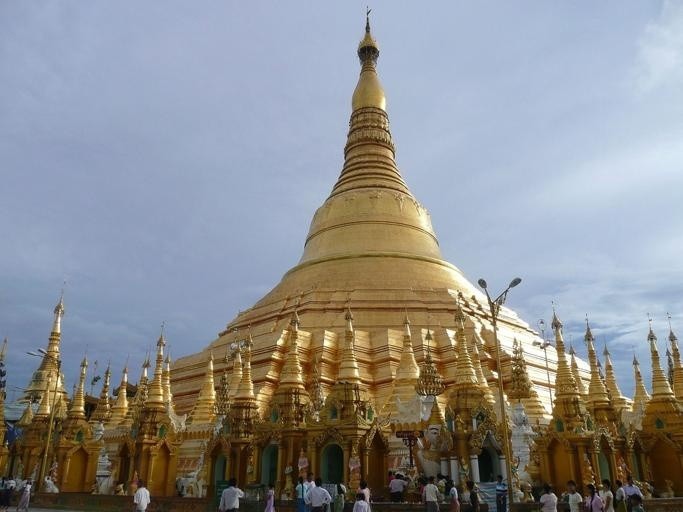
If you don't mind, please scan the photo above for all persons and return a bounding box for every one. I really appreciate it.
[0,469,657,511]
[417,423,443,480]
[48,454,58,482]
[348,446,362,490]
[297,448,309,483]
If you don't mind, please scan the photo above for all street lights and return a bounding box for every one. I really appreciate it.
[478,275,524,512]
[24,349,61,492]
[531,317,552,416]
